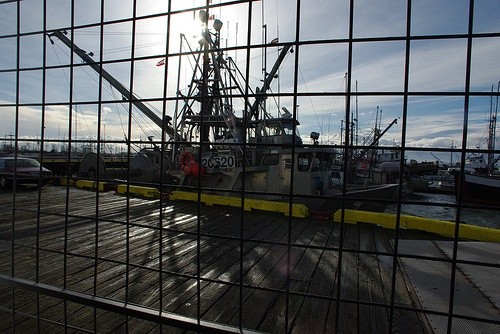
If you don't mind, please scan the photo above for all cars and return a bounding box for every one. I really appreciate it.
[0,157,51,190]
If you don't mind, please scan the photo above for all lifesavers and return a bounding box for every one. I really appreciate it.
[179,152,194,170]
[361,162,367,169]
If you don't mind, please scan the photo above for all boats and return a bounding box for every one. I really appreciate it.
[42,7,400,213]
[333,71,499,215]
